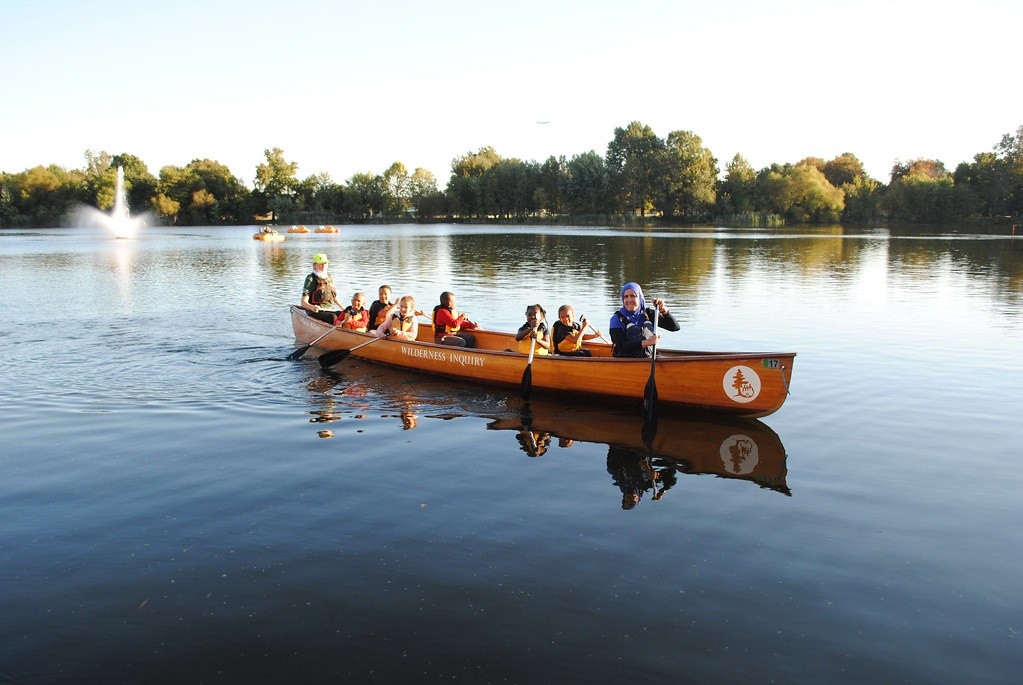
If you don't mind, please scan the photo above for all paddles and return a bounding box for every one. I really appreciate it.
[579,314,610,343]
[317,332,393,369]
[315,305,343,313]
[641,299,660,423]
[521,410,538,451]
[289,315,352,359]
[399,298,432,320]
[641,421,659,500]
[465,317,481,329]
[520,326,539,387]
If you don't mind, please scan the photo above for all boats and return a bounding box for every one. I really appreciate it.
[253,230,286,243]
[289,339,792,498]
[314,226,340,233]
[287,226,311,233]
[290,304,797,420]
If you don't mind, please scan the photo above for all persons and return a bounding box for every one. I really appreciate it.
[376,296,418,341]
[609,283,680,358]
[259,227,277,234]
[432,291,475,347]
[369,285,393,336]
[515,304,551,355]
[334,292,370,333]
[553,304,600,357]
[301,254,342,324]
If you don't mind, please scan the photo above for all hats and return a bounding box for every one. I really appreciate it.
[313,253,329,265]
